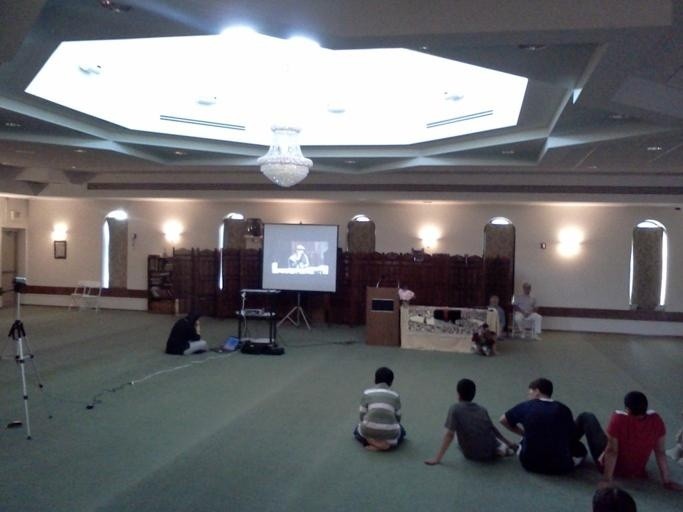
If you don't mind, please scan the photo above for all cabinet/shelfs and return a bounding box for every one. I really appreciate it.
[234,289,278,347]
[148,254,193,315]
[400,306,500,354]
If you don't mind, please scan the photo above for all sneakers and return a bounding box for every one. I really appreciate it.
[531,333,543,341]
[520,330,526,340]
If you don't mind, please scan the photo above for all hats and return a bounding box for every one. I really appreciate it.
[477,322,488,329]
[296,245,305,250]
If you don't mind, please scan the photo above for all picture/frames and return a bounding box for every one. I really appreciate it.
[54,241,67,258]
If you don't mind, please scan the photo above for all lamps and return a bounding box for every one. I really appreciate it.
[259,127,312,189]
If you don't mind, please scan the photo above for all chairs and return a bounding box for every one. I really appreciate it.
[69,281,103,314]
[511,305,534,339]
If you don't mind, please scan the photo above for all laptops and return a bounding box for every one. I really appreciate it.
[210,335,240,352]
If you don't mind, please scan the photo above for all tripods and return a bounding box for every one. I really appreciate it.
[1,319,41,438]
[276,292,313,332]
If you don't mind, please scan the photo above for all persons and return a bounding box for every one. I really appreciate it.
[471,323,500,356]
[511,282,546,343]
[570,391,683,493]
[286,244,310,268]
[589,481,637,511]
[423,378,519,466]
[352,366,405,451]
[165,308,208,355]
[499,378,576,477]
[491,295,507,340]
